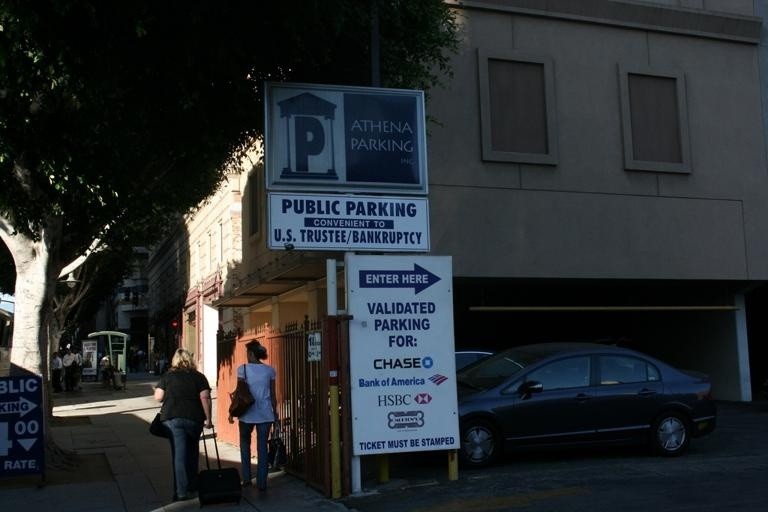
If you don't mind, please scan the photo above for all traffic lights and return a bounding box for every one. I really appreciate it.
[171,321,179,328]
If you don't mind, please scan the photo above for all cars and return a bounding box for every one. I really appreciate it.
[456,340,716,468]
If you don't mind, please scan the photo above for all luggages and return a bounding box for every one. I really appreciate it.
[198,424,242,508]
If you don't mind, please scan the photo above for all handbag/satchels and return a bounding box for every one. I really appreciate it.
[150,413,172,438]
[268,439,287,469]
[230,380,254,416]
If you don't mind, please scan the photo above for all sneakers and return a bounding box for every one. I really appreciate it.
[242,479,251,486]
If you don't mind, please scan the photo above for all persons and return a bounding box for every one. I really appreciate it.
[228,339,277,493]
[83,352,92,368]
[51,345,83,393]
[153,347,213,502]
[97,345,180,392]
